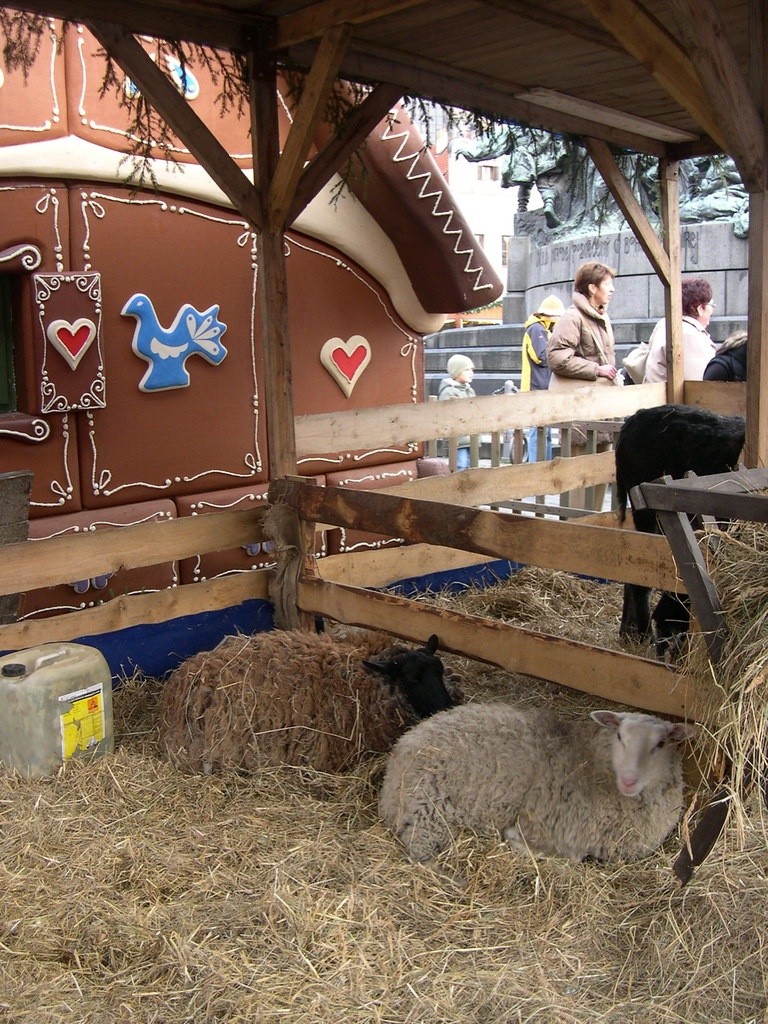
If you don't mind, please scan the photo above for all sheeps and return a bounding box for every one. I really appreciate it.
[614,400,750,661]
[164,628,703,895]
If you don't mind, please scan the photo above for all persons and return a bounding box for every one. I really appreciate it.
[454,124,566,229]
[519,296,566,464]
[546,262,616,522]
[703,331,749,383]
[438,354,479,474]
[641,278,718,385]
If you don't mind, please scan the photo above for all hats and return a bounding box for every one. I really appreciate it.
[447,354,475,380]
[538,295,565,316]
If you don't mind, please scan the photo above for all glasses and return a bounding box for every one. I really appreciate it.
[705,304,716,309]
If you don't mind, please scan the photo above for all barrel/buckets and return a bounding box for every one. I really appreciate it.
[0,642,115,778]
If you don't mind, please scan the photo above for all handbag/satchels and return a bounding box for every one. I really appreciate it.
[548,371,619,391]
[621,317,665,384]
